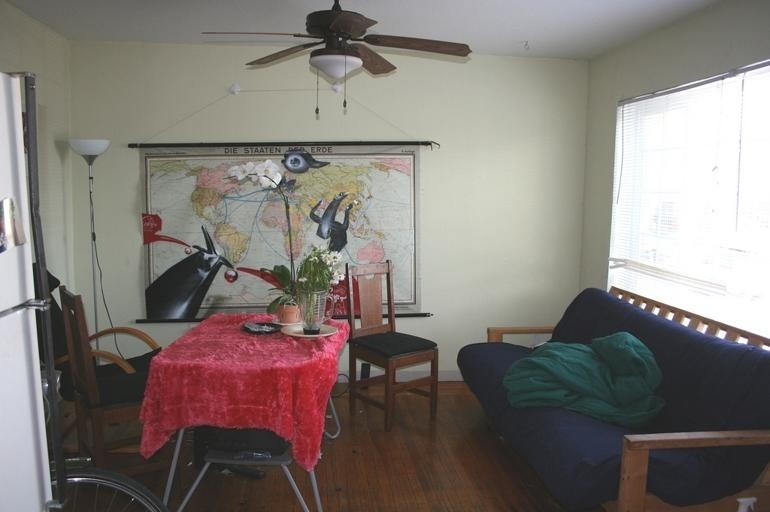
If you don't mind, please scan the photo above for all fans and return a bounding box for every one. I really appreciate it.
[199,2,473,78]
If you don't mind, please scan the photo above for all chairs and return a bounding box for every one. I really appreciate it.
[57,285,162,456]
[347,257,439,433]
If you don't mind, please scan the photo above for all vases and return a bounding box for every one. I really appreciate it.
[293,289,332,335]
[280,302,300,322]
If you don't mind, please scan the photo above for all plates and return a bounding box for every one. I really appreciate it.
[272,318,305,326]
[280,325,337,337]
[243,322,282,333]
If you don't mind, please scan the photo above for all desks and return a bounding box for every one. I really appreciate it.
[138,312,351,512]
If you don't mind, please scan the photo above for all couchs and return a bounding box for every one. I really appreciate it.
[456,283,770,512]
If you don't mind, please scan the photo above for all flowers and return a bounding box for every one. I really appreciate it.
[221,158,301,315]
[294,243,344,326]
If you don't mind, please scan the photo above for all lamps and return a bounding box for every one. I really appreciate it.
[66,137,114,366]
[307,40,366,115]
